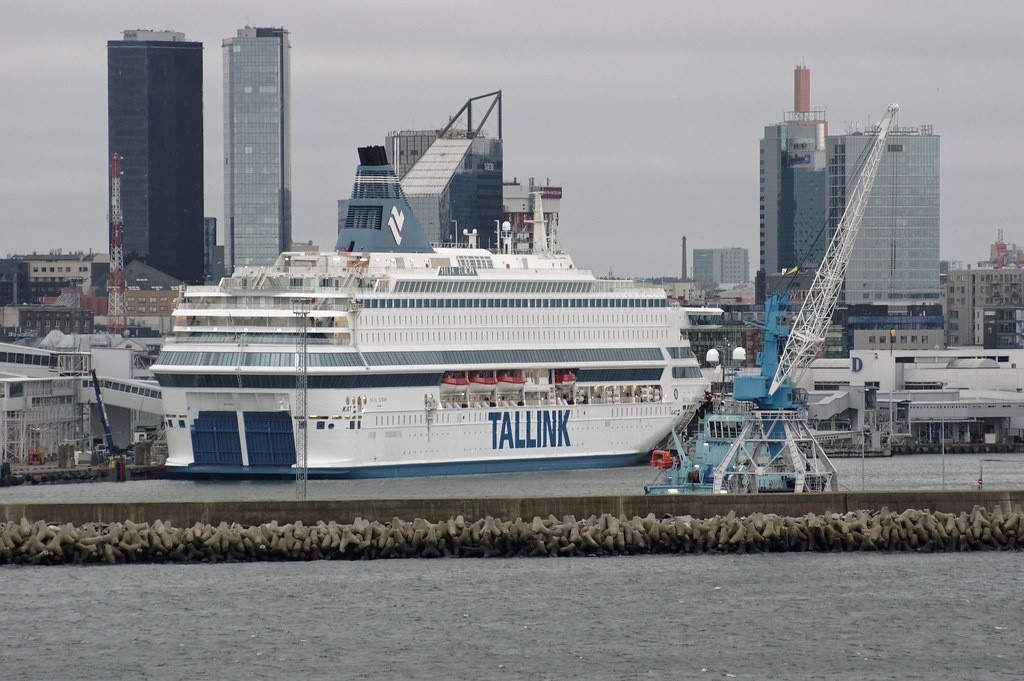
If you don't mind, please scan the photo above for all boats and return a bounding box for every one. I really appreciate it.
[467,375,499,394]
[497,375,525,394]
[439,377,469,395]
[651,449,682,470]
[549,372,578,386]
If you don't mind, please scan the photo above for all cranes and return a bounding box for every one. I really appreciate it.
[625,101,899,497]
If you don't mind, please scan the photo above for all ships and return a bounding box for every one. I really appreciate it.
[148,189,714,483]
